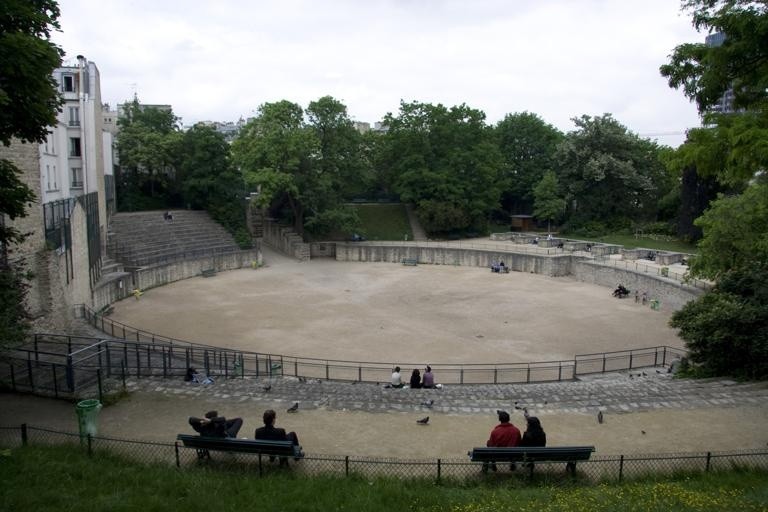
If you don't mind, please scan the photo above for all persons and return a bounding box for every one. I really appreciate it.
[486,410,521,473]
[521,417,546,467]
[253,407,305,464]
[390,366,406,389]
[422,365,434,389]
[183,365,198,382]
[410,368,421,389]
[188,408,243,440]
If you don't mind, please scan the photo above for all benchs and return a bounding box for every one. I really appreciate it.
[399,258,416,266]
[108,206,243,269]
[467,442,595,477]
[175,433,305,478]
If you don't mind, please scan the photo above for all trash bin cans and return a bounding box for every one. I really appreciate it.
[650,300,659,310]
[405,233,409,241]
[74,399,103,448]
[661,268,669,277]
[252,262,257,269]
